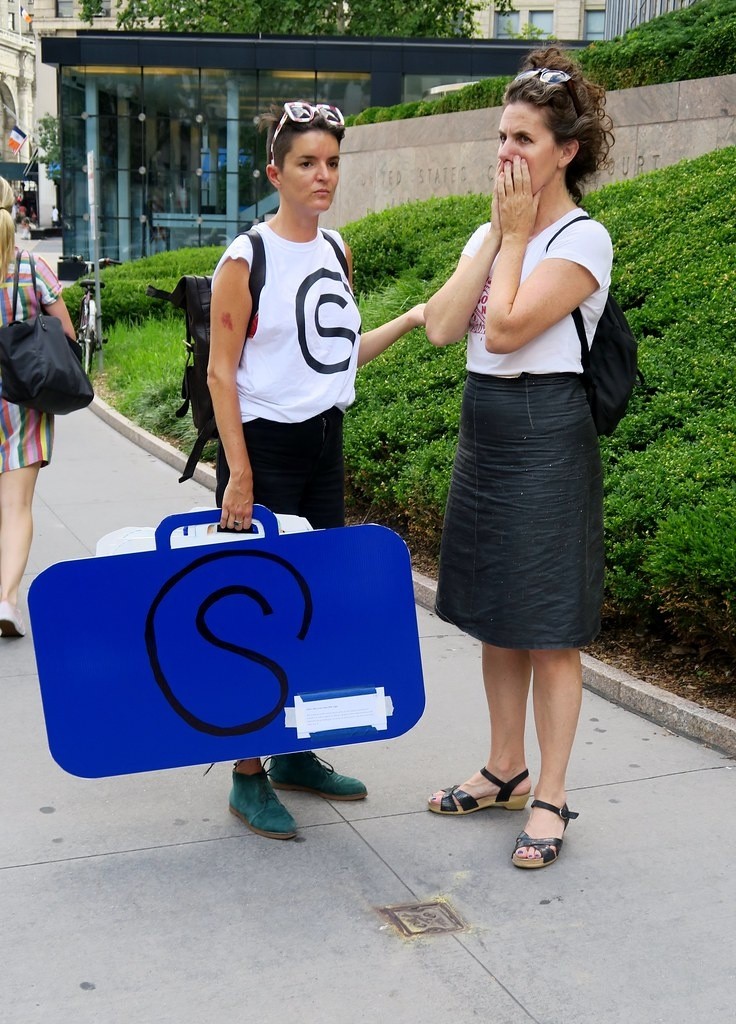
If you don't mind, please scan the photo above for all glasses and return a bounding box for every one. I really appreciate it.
[512,66,586,119]
[268,102,345,166]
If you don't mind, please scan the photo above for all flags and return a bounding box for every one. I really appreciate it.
[6,125,27,154]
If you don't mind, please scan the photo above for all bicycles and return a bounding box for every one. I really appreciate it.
[58,255,122,375]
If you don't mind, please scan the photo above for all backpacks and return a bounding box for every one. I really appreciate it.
[146,228,349,483]
[545,215,639,439]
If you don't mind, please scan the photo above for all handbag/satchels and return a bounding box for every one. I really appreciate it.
[0,249,96,415]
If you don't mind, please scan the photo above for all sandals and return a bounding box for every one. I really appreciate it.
[427,766,531,815]
[511,800,580,868]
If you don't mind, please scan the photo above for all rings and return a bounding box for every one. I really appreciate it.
[234,521,243,527]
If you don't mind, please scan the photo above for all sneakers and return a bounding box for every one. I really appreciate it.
[0,601,26,639]
[269,751,368,800]
[228,757,298,840]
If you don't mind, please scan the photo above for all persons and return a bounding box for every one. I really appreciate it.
[208,102,425,839]
[423,48,611,873]
[51,205,59,228]
[0,176,75,639]
